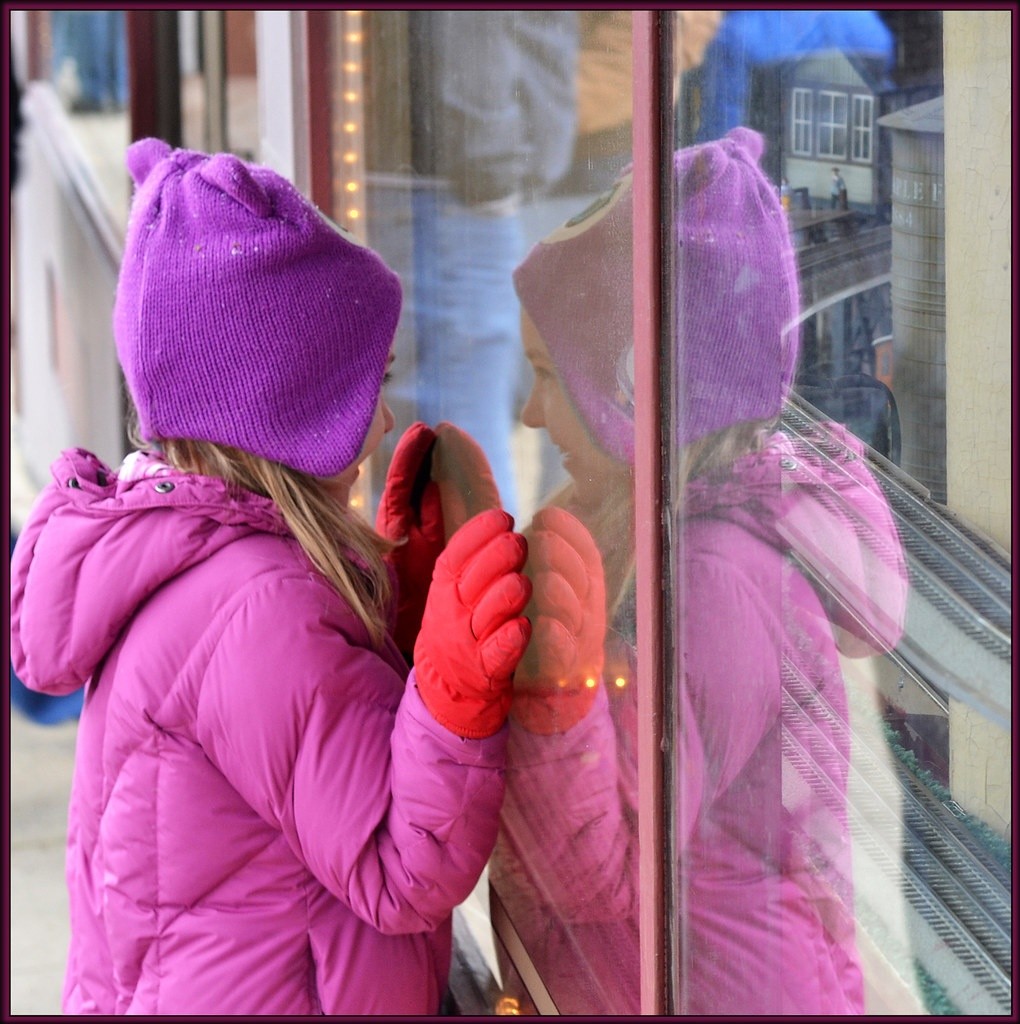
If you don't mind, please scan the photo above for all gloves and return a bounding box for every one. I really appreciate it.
[412,512,530,740]
[510,507,607,736]
[432,419,501,544]
[373,423,449,654]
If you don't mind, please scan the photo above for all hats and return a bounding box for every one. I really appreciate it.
[113,137,404,479]
[511,124,800,469]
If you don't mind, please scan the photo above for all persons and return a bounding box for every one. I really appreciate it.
[357,10,896,535]
[830,168,847,210]
[429,126,909,1016]
[778,178,792,209]
[10,136,534,1016]
